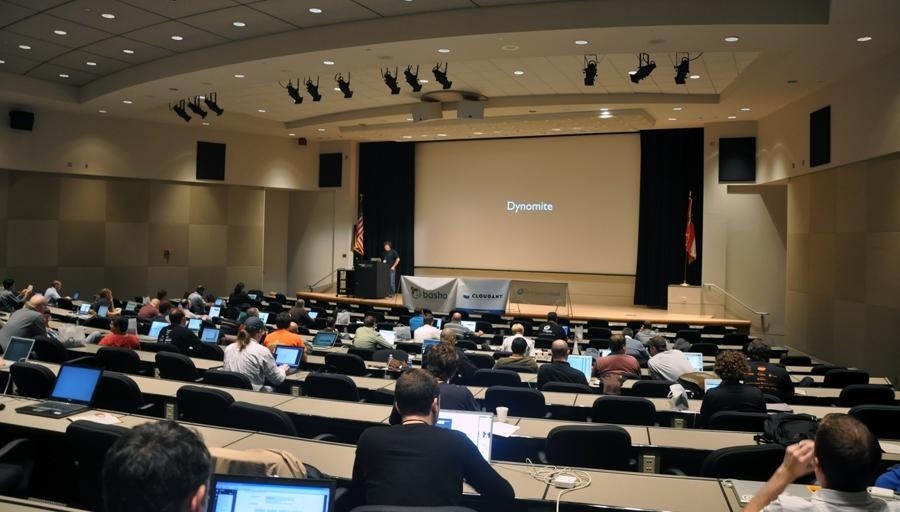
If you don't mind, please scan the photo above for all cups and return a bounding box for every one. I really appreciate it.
[495,407,509,424]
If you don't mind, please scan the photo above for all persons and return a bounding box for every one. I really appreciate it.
[99,419,215,511]
[379,240,402,296]
[352,367,515,512]
[2,275,900,428]
[740,412,900,511]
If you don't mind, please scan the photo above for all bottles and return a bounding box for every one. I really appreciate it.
[407,359,413,369]
[388,354,394,364]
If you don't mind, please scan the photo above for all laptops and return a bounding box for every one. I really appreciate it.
[703,379,746,391]
[437,410,491,469]
[16,365,104,418]
[206,475,336,512]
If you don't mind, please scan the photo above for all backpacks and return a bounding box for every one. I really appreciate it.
[753,412,819,447]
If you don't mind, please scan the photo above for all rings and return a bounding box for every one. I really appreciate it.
[798,441,803,448]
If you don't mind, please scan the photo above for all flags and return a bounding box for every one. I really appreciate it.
[350,193,365,258]
[685,190,698,264]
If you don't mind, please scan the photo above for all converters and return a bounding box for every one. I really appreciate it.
[557,475,576,489]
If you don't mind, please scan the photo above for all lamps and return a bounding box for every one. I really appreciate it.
[380,62,453,95]
[278,71,353,104]
[580,51,704,86]
[168,91,224,122]
